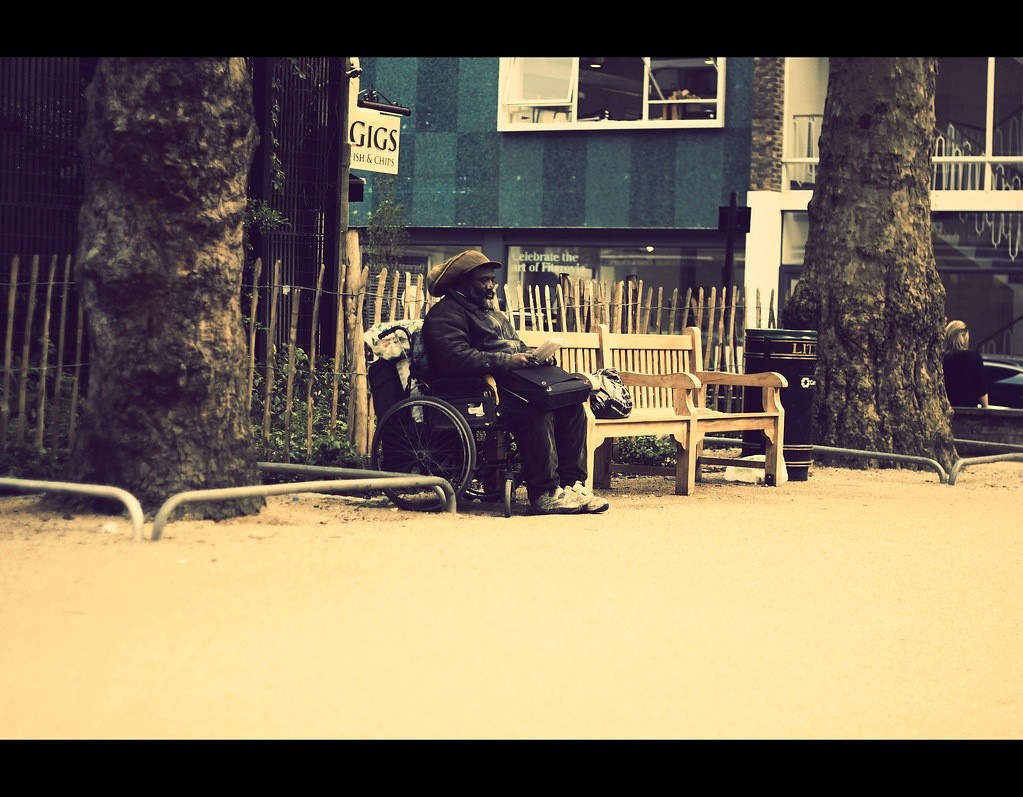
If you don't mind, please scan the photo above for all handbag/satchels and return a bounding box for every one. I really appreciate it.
[590,366,632,420]
[499,358,590,412]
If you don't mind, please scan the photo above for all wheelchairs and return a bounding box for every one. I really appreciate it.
[368,325,541,518]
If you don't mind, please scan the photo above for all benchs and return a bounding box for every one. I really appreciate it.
[485,319,788,498]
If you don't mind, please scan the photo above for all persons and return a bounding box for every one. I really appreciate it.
[944,320,988,408]
[423,250,609,514]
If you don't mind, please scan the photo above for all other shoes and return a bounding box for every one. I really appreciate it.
[531,486,583,515]
[562,481,609,514]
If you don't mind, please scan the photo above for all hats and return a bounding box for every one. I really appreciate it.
[427,249,502,298]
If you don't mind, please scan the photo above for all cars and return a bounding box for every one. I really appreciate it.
[981,355,1023,410]
